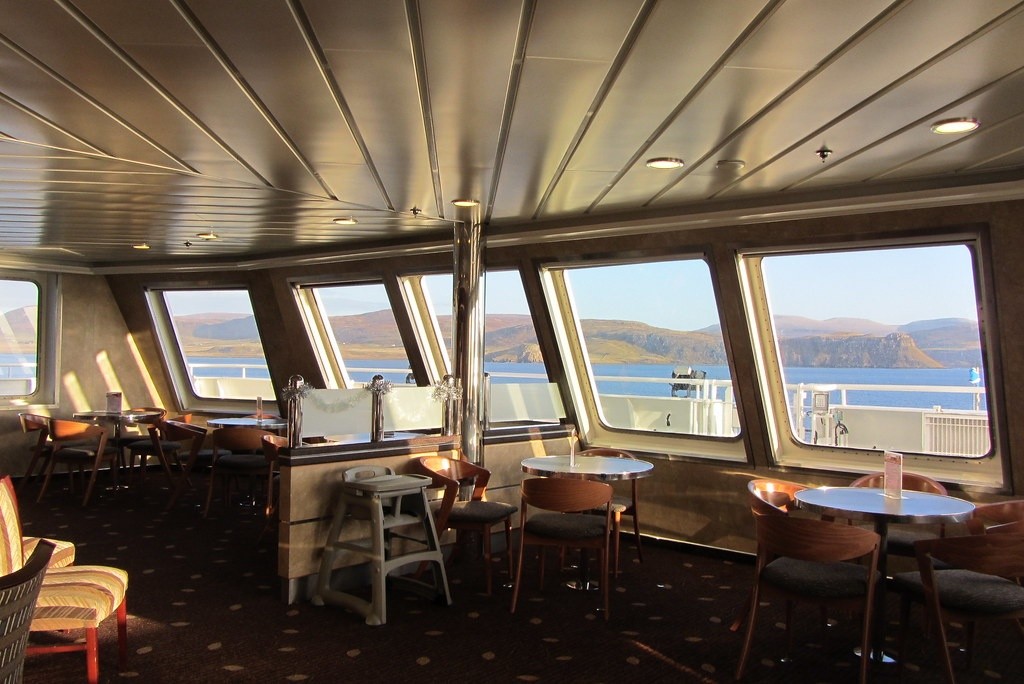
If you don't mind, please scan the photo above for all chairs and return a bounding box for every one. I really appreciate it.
[1,408,1024,684]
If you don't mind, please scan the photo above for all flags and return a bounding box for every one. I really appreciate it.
[969,367,982,384]
